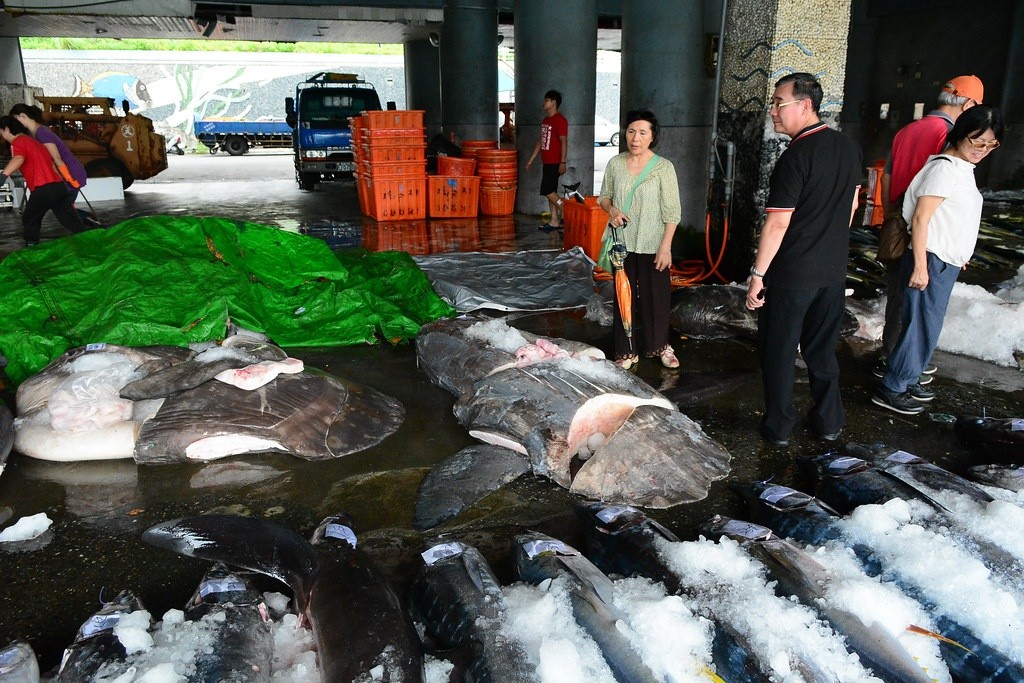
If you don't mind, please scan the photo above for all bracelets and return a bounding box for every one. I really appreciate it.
[560,162,566,163]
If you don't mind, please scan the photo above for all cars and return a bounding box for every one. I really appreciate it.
[594,114,620,147]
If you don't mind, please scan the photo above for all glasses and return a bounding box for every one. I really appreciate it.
[966,134,1001,150]
[768,97,811,115]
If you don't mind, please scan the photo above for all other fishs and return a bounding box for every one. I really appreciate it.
[0,441,1023,683]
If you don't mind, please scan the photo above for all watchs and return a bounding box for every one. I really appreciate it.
[749,263,764,277]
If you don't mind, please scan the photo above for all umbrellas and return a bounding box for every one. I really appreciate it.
[608,218,632,350]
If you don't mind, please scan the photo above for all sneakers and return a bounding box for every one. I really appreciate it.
[871,385,922,414]
[906,382,935,401]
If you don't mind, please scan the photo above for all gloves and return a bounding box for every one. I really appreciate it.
[58,165,80,189]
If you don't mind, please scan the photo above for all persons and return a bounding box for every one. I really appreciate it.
[871,104,1004,415]
[0,115,88,245]
[873,75,984,384]
[595,109,681,369]
[745,72,862,446]
[526,89,568,230]
[8,103,109,230]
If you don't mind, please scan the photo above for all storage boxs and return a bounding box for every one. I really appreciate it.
[561,195,612,262]
[346,110,482,221]
[862,160,886,227]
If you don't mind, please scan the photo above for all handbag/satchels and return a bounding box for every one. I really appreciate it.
[599,238,615,274]
[876,210,909,259]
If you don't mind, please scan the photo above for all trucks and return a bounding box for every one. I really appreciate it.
[283,71,384,192]
[192,112,295,157]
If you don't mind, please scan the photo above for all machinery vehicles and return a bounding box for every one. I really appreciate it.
[31,95,169,192]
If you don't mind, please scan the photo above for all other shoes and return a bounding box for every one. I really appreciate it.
[819,426,842,441]
[660,344,679,368]
[770,434,789,446]
[615,352,639,371]
[873,355,934,385]
[923,363,938,375]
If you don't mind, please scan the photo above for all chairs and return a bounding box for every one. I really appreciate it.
[305,100,324,120]
[348,98,366,117]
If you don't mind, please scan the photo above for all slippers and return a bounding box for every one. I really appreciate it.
[540,223,560,229]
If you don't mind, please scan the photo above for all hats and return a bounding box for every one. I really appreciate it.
[944,75,986,104]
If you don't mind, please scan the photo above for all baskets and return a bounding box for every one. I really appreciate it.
[437,139,518,216]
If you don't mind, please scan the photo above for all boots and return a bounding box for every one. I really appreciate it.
[84,213,108,228]
